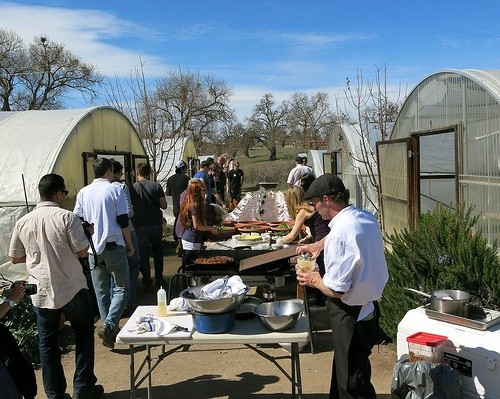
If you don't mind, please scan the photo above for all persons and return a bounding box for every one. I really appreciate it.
[9,173,104,399]
[282,185,332,306]
[296,173,389,399]
[74,158,135,349]
[286,155,314,220]
[0,283,37,399]
[110,153,244,316]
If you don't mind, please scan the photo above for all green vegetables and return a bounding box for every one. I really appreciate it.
[216,225,229,230]
[277,223,288,228]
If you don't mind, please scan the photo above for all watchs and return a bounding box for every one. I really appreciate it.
[4,299,15,308]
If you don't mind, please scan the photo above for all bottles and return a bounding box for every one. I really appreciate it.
[156,285,167,317]
[262,285,276,302]
[275,236,283,250]
[146,318,154,332]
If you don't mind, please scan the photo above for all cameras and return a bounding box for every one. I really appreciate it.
[11,281,37,295]
[80,217,89,227]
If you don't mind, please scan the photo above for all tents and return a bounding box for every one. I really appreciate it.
[307,150,327,179]
[141,137,198,190]
[322,122,394,220]
[0,105,151,283]
[376,69,500,260]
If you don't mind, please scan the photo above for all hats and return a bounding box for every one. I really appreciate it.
[175,160,190,168]
[304,172,347,201]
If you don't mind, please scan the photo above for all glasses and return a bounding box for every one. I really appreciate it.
[58,190,69,197]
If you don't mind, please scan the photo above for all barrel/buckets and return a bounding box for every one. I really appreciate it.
[406,332,448,364]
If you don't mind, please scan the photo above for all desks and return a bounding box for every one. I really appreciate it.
[116,301,313,399]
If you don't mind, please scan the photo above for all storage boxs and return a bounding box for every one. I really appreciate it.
[409,331,448,363]
[396,301,500,399]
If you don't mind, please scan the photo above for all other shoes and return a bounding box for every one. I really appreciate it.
[156,279,166,286]
[126,303,138,309]
[72,384,104,399]
[98,324,115,349]
[142,277,152,286]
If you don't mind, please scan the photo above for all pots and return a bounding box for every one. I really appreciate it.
[403,287,472,318]
[180,284,250,314]
[188,306,240,334]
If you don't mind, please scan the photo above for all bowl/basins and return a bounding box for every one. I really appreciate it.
[269,225,293,236]
[236,219,266,226]
[252,300,305,331]
[234,295,263,319]
[238,225,271,235]
[220,221,237,226]
[269,221,295,227]
[211,226,235,240]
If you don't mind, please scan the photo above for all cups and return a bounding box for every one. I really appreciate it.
[296,257,316,272]
[231,232,290,250]
[239,190,282,222]
